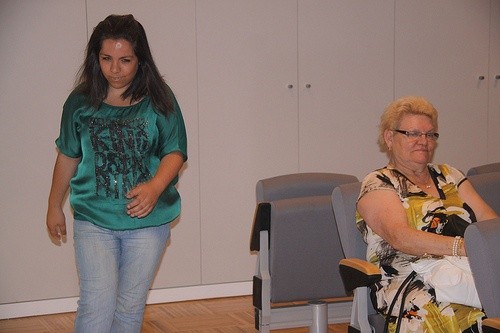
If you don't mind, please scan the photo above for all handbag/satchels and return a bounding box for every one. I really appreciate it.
[383,203,482,333]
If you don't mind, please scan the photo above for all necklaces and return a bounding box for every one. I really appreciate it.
[423,181,433,190]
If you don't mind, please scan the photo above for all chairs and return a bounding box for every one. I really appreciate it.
[248,162,500,333]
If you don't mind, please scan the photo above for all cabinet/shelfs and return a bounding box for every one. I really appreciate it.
[0,0,500,320]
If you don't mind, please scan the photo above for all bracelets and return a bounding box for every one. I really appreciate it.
[452,235,464,257]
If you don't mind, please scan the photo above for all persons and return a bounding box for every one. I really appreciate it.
[47,13,189,333]
[355,96,500,333]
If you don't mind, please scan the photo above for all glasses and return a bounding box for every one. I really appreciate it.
[395,129,439,142]
